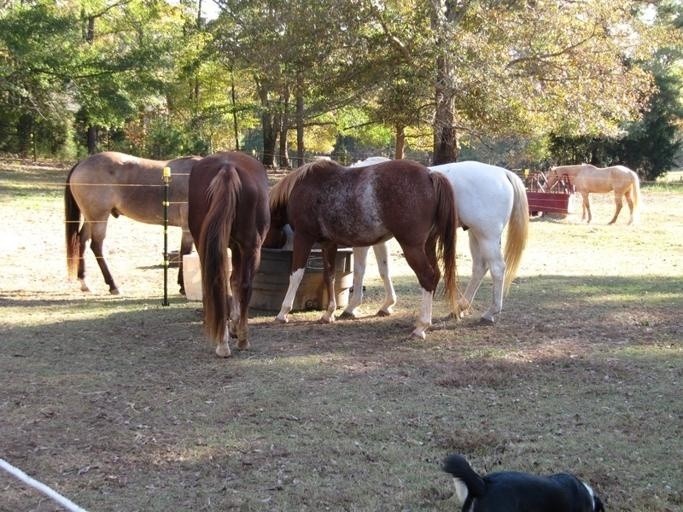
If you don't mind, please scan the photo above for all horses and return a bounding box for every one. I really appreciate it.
[263,158,474,342]
[186,150,272,358]
[335,156,530,327]
[544,165,640,225]
[64,151,204,296]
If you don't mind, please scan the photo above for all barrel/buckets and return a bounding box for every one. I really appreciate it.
[183,252,206,302]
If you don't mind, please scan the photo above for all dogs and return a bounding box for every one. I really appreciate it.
[441,452,606,512]
